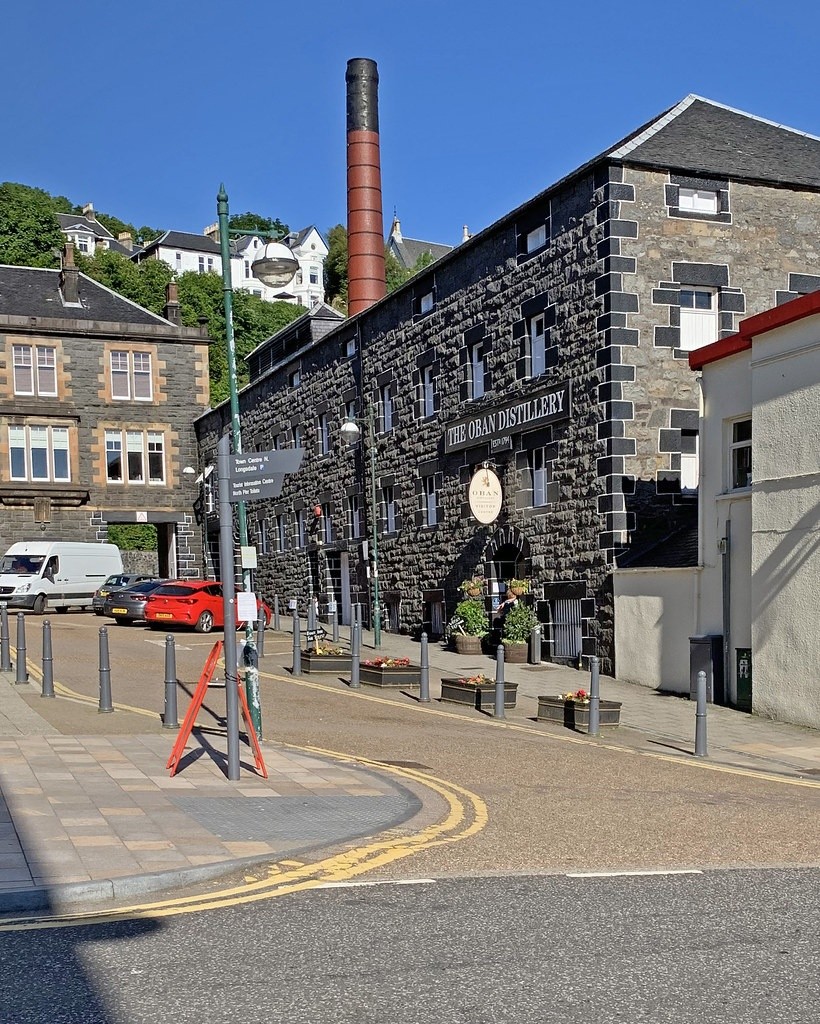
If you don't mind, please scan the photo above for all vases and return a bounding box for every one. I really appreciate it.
[537,696,622,728]
[358,662,421,689]
[439,678,519,709]
[467,589,481,595]
[300,651,353,675]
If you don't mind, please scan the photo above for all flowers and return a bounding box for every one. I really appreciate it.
[365,656,410,668]
[456,578,484,591]
[303,646,342,654]
[459,674,490,686]
[564,690,589,702]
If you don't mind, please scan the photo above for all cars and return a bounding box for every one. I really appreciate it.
[145,581,271,634]
[93,574,161,615]
[105,582,164,625]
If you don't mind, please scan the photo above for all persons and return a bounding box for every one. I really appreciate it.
[497,589,519,618]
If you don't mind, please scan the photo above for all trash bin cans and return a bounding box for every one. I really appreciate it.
[688,633,724,702]
[734,647,752,710]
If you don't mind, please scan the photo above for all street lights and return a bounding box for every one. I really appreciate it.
[214,181,301,739]
[341,407,383,650]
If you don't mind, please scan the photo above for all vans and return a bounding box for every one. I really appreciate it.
[0,542,123,613]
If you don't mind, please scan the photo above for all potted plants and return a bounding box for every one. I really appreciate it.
[510,579,530,596]
[500,598,541,664]
[451,595,490,654]
